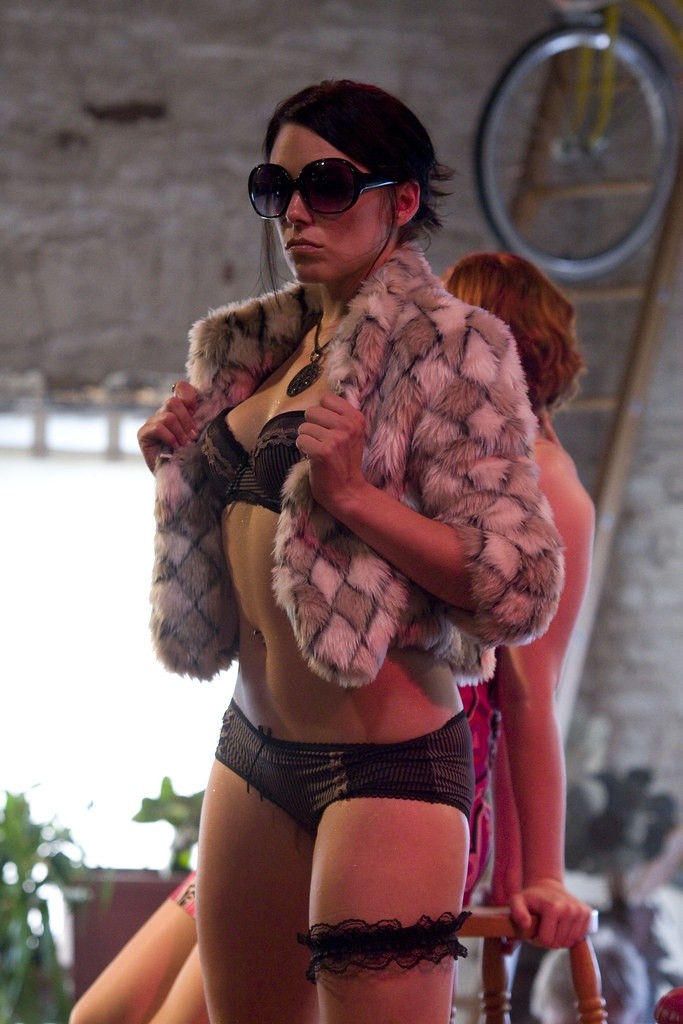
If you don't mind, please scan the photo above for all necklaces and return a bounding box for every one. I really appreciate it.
[287,317,338,398]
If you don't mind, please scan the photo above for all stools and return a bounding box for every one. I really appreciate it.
[444,907,608,1024]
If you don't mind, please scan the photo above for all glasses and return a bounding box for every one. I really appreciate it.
[248,157,407,218]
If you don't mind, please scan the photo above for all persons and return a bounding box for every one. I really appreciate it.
[136,77,569,1023]
[56,253,599,1023]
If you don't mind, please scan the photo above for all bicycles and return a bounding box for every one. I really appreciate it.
[473,0,683,283]
